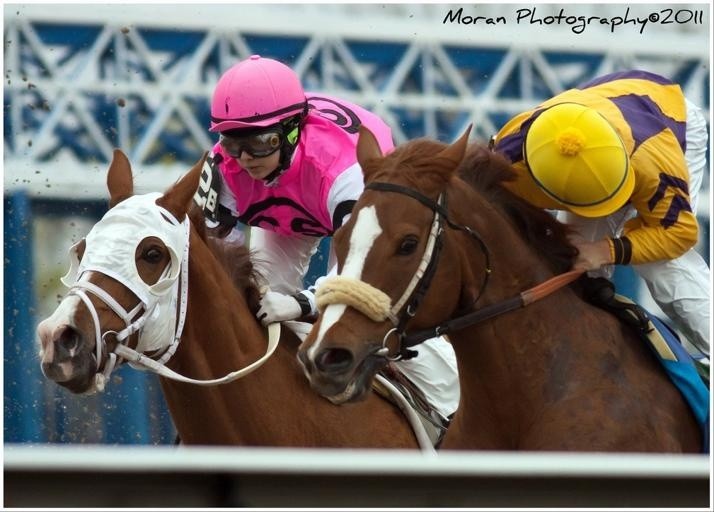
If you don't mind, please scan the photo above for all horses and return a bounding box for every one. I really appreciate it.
[37,148,449,508]
[296,122,710,508]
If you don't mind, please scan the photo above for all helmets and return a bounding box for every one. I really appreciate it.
[522,100,637,221]
[208,54,308,133]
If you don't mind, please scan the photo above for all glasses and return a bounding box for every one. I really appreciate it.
[218,126,299,158]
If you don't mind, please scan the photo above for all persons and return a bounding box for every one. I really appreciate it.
[483,68,711,361]
[187,52,461,432]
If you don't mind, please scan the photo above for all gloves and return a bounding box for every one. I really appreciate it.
[255,289,311,328]
[567,236,614,273]
